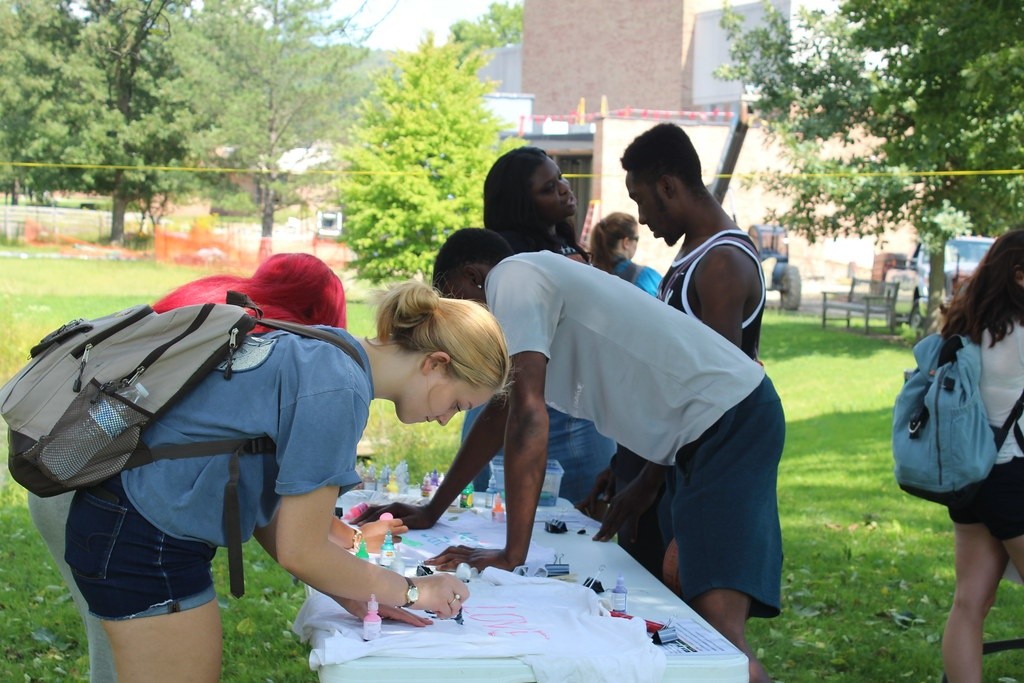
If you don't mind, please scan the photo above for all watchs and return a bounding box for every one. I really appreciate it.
[402,577,419,608]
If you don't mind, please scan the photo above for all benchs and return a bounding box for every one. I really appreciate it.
[821,276,901,335]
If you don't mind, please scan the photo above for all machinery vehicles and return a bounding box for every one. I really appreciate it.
[749,224,801,310]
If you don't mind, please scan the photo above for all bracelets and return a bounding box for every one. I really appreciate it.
[353,528,362,547]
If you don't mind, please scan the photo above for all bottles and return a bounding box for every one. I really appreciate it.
[611,572,627,613]
[421,469,445,505]
[459,482,474,508]
[455,563,471,583]
[354,529,406,578]
[356,460,410,496]
[341,502,370,522]
[39,382,151,481]
[363,594,382,641]
[484,473,505,524]
[380,512,394,522]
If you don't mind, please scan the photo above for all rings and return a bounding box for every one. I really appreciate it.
[449,599,456,605]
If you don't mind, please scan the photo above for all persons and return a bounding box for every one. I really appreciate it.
[349,227,787,683]
[942,230,1024,683]
[62,277,510,683]
[461,147,619,520]
[30,252,408,683]
[591,212,663,298]
[577,124,767,579]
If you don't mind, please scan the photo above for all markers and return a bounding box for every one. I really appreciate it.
[611,611,667,633]
[416,565,465,626]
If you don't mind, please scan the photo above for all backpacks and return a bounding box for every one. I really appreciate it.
[892,325,1024,506]
[0,291,363,497]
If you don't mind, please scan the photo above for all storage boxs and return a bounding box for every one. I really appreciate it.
[488,456,565,506]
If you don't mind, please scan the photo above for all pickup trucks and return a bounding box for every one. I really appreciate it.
[884,235,998,329]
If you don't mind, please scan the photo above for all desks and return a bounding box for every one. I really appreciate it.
[291,483,751,683]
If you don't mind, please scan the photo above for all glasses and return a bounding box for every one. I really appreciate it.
[618,235,638,244]
